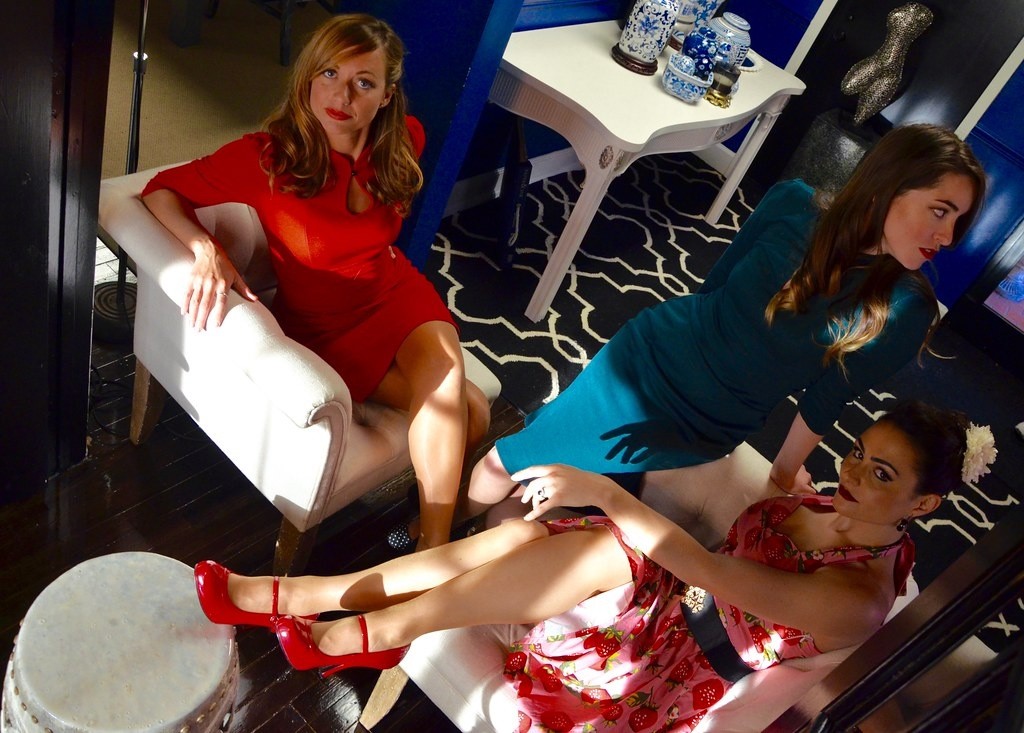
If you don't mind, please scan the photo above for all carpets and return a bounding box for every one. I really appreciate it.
[429,153,1024,655]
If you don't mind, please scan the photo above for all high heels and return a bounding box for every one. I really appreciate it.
[194,560,320,632]
[275,614,411,679]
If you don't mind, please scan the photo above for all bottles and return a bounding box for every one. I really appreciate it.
[696,32,718,59]
[682,27,709,60]
[712,41,731,65]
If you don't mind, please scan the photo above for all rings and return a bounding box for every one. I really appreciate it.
[538,486,547,499]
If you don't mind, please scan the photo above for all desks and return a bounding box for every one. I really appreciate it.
[494,13,806,324]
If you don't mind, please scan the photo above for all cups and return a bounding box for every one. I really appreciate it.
[704,62,742,108]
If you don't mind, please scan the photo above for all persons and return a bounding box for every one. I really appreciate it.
[385,125,988,552]
[136,15,489,553]
[195,398,997,733]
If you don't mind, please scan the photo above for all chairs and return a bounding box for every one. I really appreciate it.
[350,443,1024,733]
[0,554,238,733]
[94,158,502,573]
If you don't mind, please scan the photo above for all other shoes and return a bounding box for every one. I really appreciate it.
[386,514,419,551]
